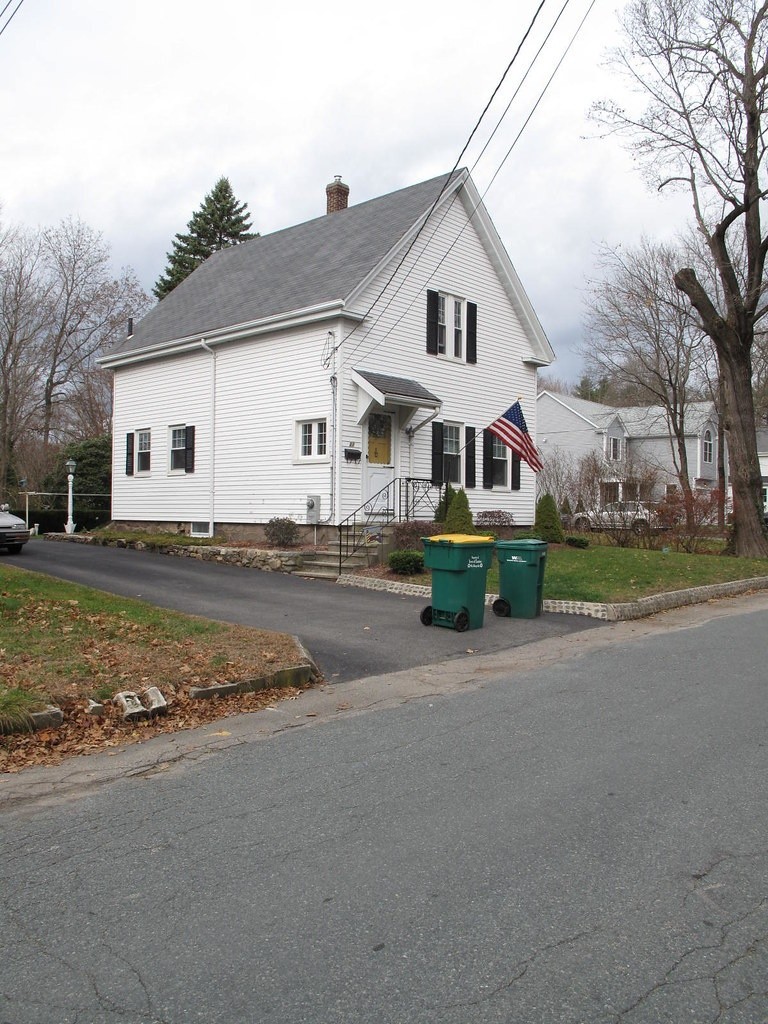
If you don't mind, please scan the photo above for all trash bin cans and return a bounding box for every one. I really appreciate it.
[420,534,497,632]
[491,539,548,620]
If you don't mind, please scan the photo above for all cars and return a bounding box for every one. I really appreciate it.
[567,501,675,536]
[0,503,31,554]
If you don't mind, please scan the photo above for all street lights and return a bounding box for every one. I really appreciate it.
[65,457,77,535]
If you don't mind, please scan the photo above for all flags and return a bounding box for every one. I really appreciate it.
[482,400,543,473]
